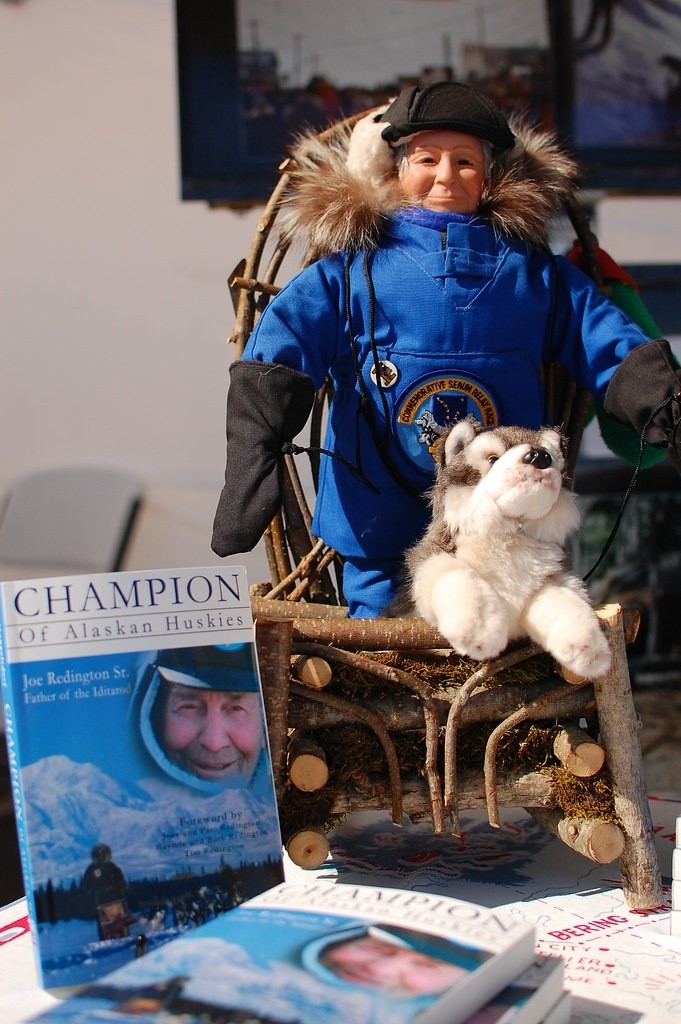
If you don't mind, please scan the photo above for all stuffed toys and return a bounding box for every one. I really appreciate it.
[393,415,614,680]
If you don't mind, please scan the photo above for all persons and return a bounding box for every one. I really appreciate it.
[78,842,131,941]
[129,642,271,799]
[297,920,483,1004]
[208,81,681,618]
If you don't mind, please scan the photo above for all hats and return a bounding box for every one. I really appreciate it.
[154,642,260,692]
[365,922,490,974]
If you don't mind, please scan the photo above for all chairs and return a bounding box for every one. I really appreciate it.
[225,97,664,915]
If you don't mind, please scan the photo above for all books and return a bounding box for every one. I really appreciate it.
[0,566,286,989]
[31,879,574,1024]
